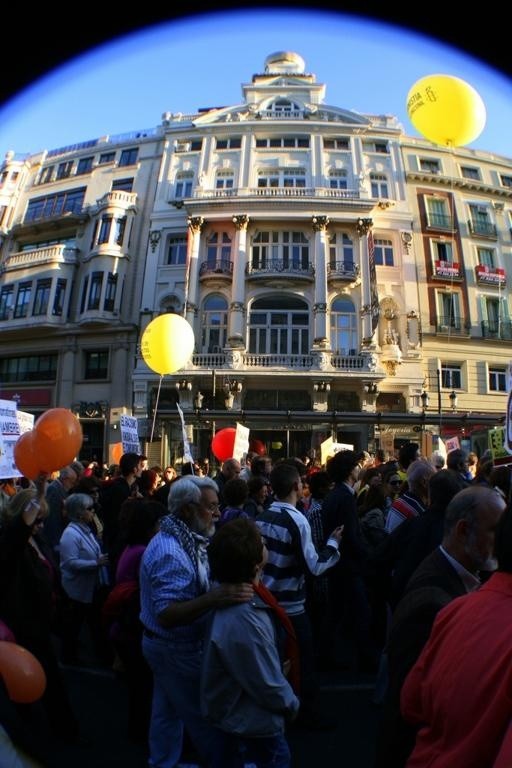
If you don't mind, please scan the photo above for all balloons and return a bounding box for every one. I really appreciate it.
[30,406,86,473]
[404,72,489,149]
[139,312,196,378]
[11,429,49,482]
[209,425,238,463]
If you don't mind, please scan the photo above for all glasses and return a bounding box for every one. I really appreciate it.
[86,505,93,511]
[390,480,403,486]
[34,517,44,525]
[261,535,267,546]
[207,501,222,512]
